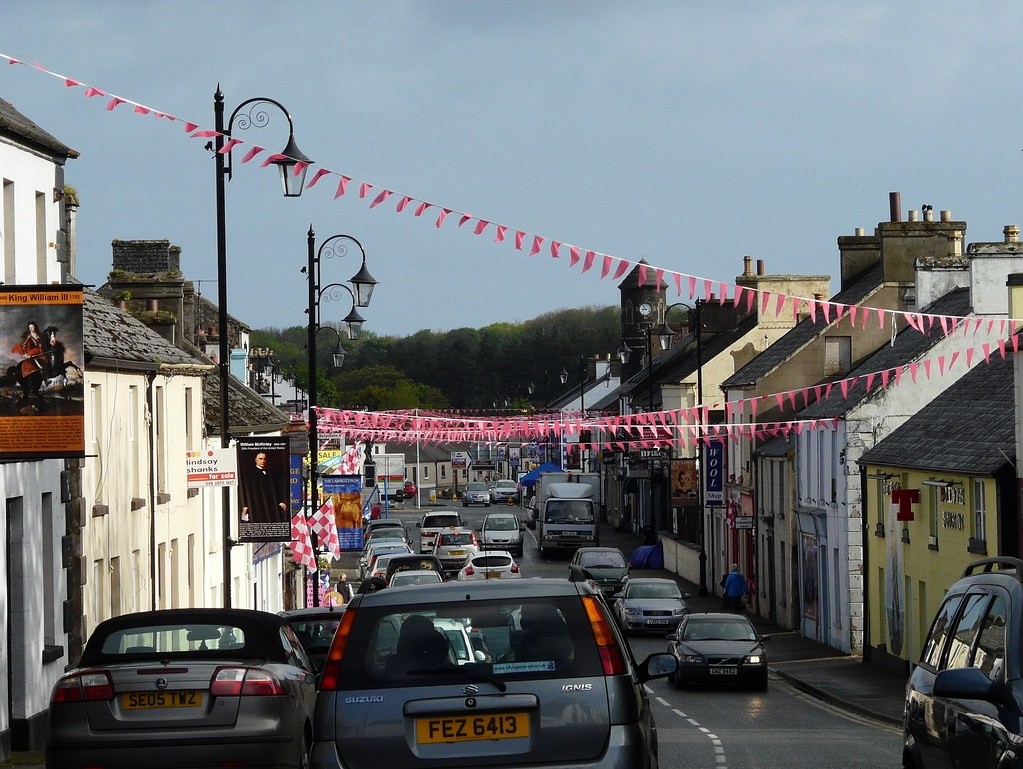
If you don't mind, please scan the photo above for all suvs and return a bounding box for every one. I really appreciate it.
[901,557,1023,769]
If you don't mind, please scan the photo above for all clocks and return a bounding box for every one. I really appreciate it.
[639,302,652,316]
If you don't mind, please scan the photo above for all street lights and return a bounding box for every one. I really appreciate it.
[257,354,273,396]
[206,80,315,647]
[271,361,296,405]
[602,359,631,534]
[527,368,549,462]
[618,322,657,546]
[560,351,585,475]
[298,221,381,640]
[657,302,708,597]
[503,391,523,471]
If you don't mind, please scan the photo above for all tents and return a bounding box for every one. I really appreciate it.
[519,461,565,520]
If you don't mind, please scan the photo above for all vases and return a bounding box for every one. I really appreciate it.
[207,326,216,336]
[146,300,159,311]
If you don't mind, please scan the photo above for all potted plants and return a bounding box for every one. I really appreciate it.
[110,290,133,309]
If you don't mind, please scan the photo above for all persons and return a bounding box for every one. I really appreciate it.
[21,321,42,349]
[334,572,354,604]
[240,452,287,523]
[725,564,749,614]
[673,471,692,497]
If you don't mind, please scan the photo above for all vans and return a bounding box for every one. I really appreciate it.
[402,480,416,499]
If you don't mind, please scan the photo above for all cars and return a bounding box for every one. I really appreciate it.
[462,479,520,507]
[275,510,526,674]
[666,612,772,691]
[524,496,537,530]
[569,547,634,598]
[45,607,322,769]
[613,577,693,637]
[308,566,682,769]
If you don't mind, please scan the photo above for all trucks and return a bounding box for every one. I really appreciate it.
[371,452,407,502]
[532,472,602,560]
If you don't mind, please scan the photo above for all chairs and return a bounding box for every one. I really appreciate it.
[515,626,573,661]
[385,650,447,680]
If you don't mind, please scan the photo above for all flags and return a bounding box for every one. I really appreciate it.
[332,444,360,474]
[307,497,341,561]
[284,508,317,574]
